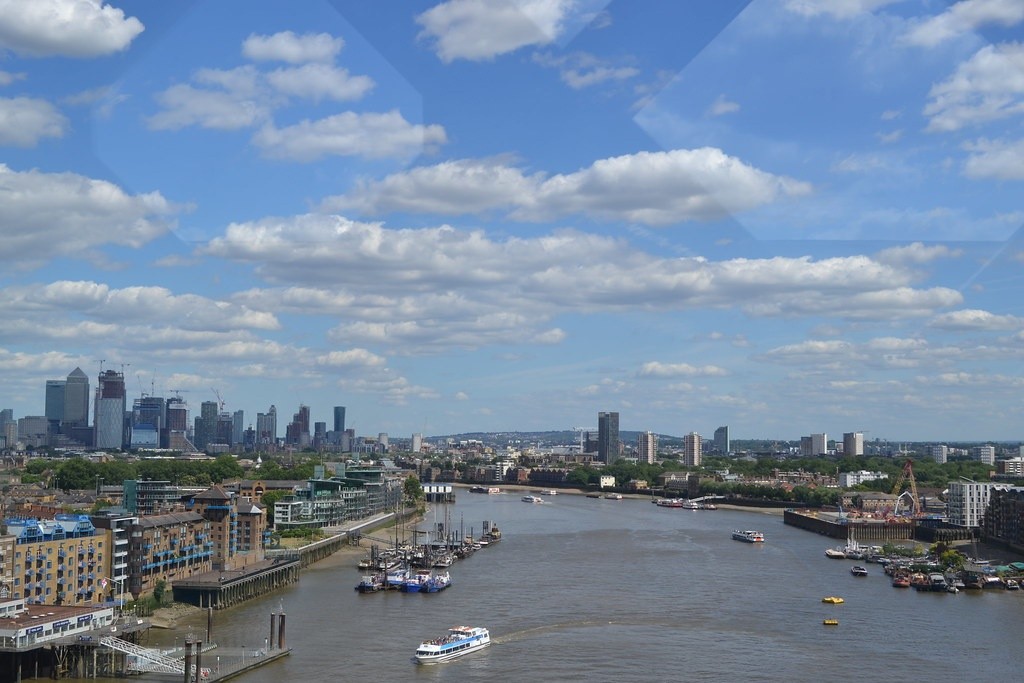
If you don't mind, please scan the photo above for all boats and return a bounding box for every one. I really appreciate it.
[825,548,846,559]
[732,529,764,542]
[605,492,622,500]
[824,619,838,625]
[470,485,489,493]
[353,519,503,593]
[851,565,868,575]
[415,625,491,665]
[867,553,1024,593]
[521,495,544,503]
[541,489,559,495]
[656,498,718,510]
[822,597,844,603]
